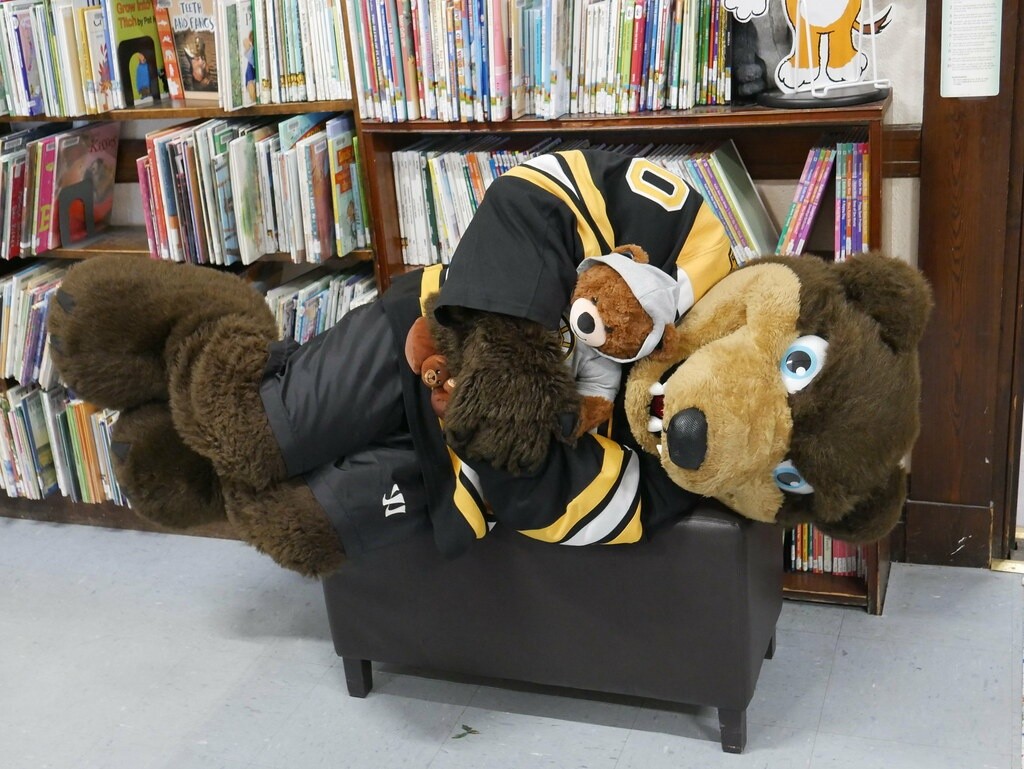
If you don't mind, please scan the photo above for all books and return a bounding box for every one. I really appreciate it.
[782,523,868,581]
[134,114,372,268]
[0,0,355,118]
[0,121,123,261]
[391,133,589,267]
[345,0,734,124]
[0,261,75,390]
[774,126,870,267]
[0,382,133,511]
[236,260,378,347]
[587,138,779,268]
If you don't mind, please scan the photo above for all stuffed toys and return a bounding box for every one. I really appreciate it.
[49,146,931,574]
[401,243,700,440]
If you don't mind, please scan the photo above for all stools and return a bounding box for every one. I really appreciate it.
[320,498,785,757]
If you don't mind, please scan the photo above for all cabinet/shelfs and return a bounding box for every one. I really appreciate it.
[357,108,902,619]
[0,0,381,541]
